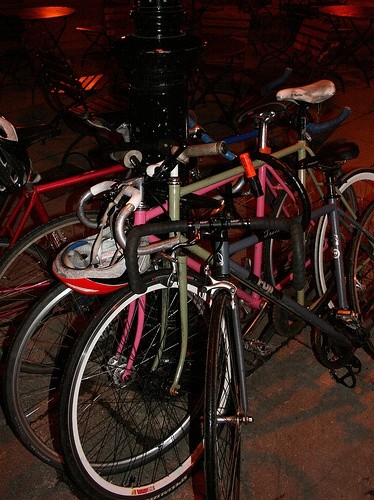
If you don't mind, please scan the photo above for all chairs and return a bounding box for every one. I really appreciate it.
[1,0,374,135]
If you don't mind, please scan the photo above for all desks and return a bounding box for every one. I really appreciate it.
[183,36,247,131]
[18,5,77,105]
[320,5,374,87]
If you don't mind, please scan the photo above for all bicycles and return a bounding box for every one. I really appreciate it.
[0,78,374,500]
